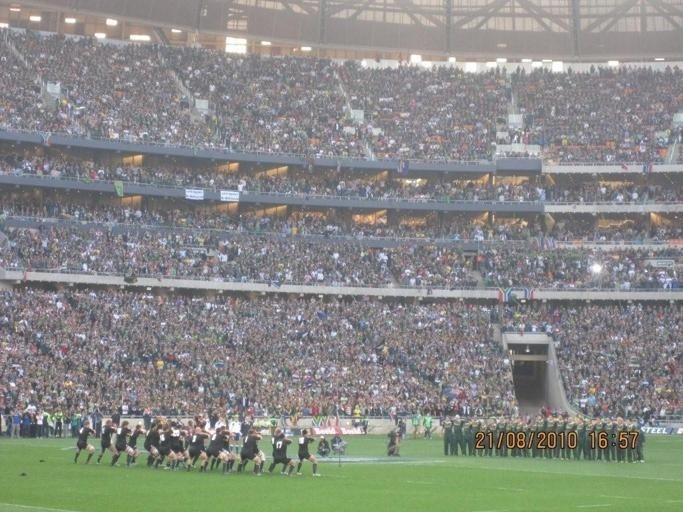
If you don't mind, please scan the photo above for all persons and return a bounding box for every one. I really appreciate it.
[0,28,682,476]
